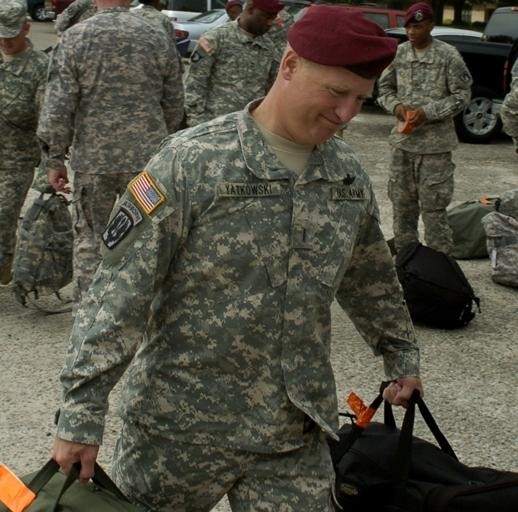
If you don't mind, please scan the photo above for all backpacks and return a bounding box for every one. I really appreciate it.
[392,241,483,332]
[9,177,76,305]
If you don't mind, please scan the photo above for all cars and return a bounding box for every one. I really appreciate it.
[27,1,75,22]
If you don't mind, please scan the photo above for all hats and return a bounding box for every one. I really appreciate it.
[0,0,32,40]
[285,3,399,81]
[252,0,286,18]
[402,2,436,29]
[224,0,243,14]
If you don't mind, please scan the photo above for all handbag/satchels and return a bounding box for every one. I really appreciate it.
[443,187,518,290]
[327,377,518,512]
[0,449,146,512]
